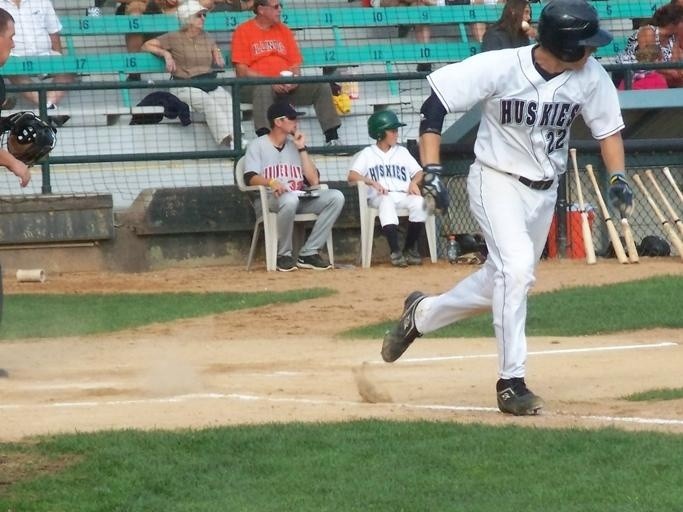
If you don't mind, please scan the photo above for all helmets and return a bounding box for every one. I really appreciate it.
[367,111,407,139]
[641,236,670,256]
[7,112,57,166]
[455,232,488,255]
[538,0,613,62]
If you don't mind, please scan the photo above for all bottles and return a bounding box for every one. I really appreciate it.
[349,72,360,99]
[445,236,461,264]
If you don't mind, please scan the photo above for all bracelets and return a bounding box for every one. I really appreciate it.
[268,178,277,187]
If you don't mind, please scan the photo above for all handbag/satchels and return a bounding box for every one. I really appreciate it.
[189,72,218,91]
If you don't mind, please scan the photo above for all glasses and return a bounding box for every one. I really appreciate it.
[196,13,207,17]
[260,4,283,9]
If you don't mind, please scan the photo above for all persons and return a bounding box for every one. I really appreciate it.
[0,7,57,379]
[361,0,683,90]
[380,0,634,416]
[244,102,345,273]
[0,0,349,159]
[348,109,426,268]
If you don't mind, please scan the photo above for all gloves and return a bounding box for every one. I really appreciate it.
[607,173,634,218]
[420,164,450,216]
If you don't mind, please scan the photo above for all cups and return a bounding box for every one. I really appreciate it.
[14,268,46,285]
[279,70,293,87]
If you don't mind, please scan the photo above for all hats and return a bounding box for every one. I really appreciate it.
[267,101,305,120]
[176,0,207,17]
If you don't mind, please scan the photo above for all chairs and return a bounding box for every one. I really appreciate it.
[358,180,437,269]
[235,155,335,272]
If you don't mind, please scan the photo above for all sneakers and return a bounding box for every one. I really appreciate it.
[381,290,426,363]
[325,139,349,157]
[406,248,421,264]
[497,379,545,415]
[391,251,408,267]
[296,254,332,271]
[277,256,298,272]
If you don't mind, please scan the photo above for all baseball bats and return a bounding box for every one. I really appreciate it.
[570,148,596,265]
[663,166,683,201]
[621,208,639,263]
[646,170,682,234]
[632,173,683,260]
[584,164,627,264]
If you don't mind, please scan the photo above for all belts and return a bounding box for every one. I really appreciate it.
[511,173,554,190]
